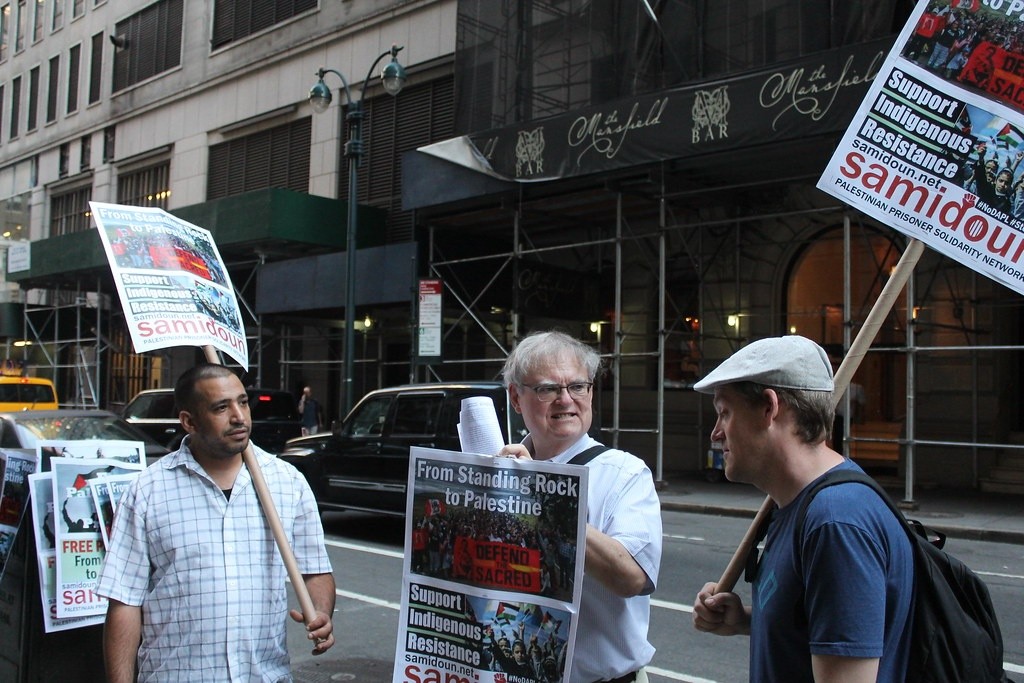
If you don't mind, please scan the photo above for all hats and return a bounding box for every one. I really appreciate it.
[693,334,836,395]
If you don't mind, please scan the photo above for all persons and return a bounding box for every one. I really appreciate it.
[902,1,1023,221]
[498,329,666,683]
[689,332,916,683]
[97,363,339,683]
[299,386,323,437]
[2,443,143,552]
[106,224,242,332]
[412,491,576,682]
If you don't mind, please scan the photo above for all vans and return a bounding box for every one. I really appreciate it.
[0,376,58,415]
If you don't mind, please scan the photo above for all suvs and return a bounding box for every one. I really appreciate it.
[114,388,301,459]
[282,381,520,530]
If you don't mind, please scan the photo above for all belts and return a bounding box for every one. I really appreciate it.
[607,671,636,683]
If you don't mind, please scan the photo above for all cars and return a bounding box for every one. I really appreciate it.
[0,409,173,533]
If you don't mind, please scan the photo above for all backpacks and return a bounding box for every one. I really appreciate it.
[792,469,1010,683]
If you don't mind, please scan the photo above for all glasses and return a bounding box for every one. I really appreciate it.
[746,510,771,584]
[517,380,597,403]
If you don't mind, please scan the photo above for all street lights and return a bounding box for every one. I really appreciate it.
[309,44,408,434]
